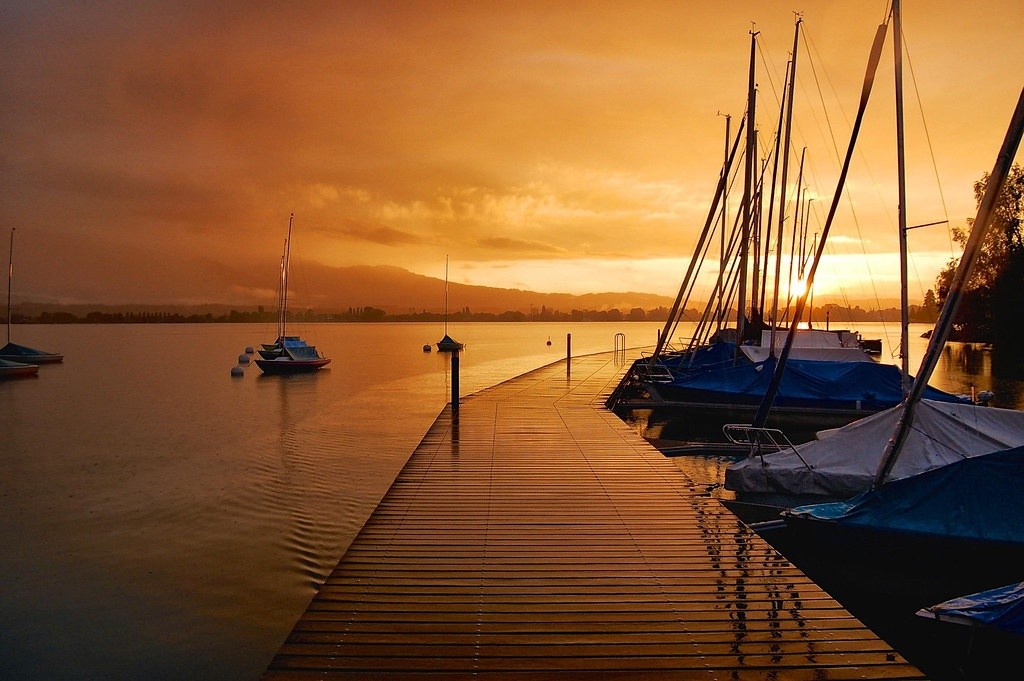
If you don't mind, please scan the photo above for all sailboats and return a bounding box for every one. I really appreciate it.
[0,226,64,365]
[620,0,1024,652]
[436,254,465,351]
[253,213,331,374]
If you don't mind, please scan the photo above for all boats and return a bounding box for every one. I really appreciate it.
[0,358,40,377]
